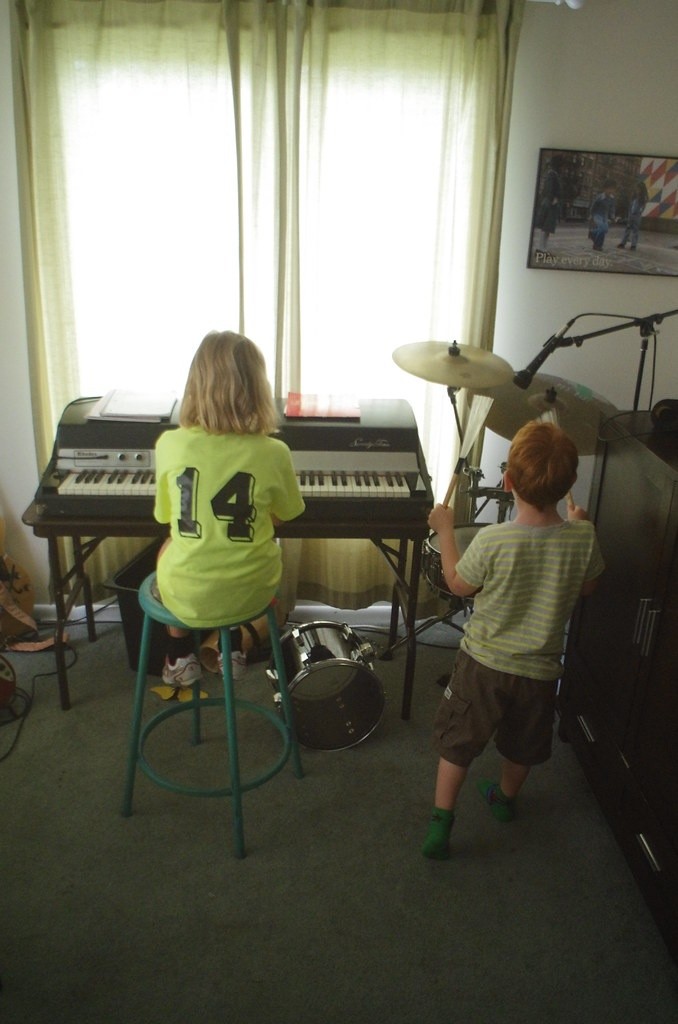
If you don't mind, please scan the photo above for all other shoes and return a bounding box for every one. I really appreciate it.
[631,246,636,249]
[594,244,602,250]
[617,244,624,249]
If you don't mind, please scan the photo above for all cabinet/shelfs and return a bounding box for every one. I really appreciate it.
[559,412,678,960]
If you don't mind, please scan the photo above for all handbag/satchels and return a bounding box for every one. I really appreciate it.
[588,215,598,241]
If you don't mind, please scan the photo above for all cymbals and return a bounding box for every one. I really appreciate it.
[467,373,629,455]
[392,341,513,388]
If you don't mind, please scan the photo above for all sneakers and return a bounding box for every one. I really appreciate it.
[163,653,201,686]
[216,641,246,680]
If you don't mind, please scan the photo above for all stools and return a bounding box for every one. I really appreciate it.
[119,571,303,858]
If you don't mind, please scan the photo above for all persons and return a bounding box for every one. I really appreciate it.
[419,421,606,860]
[536,154,567,256]
[153,330,306,687]
[617,182,649,251]
[588,177,618,251]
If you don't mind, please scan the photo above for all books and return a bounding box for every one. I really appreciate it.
[83,388,177,423]
[284,391,362,418]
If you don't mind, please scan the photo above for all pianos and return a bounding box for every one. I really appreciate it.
[21,393,434,538]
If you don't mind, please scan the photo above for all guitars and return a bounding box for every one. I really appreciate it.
[0,517,35,640]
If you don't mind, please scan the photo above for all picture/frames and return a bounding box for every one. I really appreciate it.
[526,148,678,277]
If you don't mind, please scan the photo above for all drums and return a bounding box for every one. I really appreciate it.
[420,520,498,598]
[268,620,385,752]
